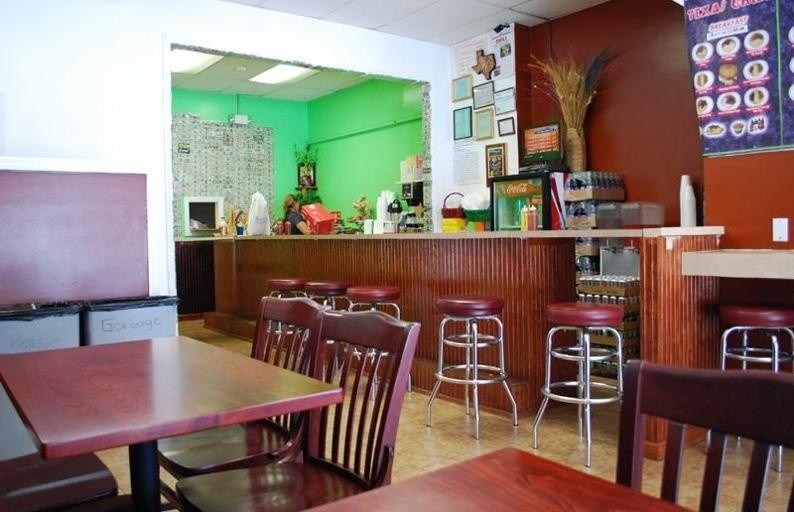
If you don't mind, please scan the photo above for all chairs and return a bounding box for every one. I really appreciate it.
[181,305,422,512]
[0,429,136,511]
[159,288,345,512]
[611,345,794,512]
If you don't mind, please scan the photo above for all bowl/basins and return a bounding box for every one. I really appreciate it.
[441,207,467,233]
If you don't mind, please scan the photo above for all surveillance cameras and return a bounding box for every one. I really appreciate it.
[494,24,502,33]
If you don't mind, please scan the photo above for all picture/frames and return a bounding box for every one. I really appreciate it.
[484,143,510,190]
[497,115,515,137]
[492,86,517,116]
[471,107,495,140]
[295,163,316,187]
[471,82,495,110]
[450,75,473,104]
[452,107,472,140]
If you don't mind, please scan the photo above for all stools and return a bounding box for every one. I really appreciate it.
[422,290,521,443]
[259,278,306,363]
[531,302,632,468]
[340,285,413,401]
[704,299,794,473]
[297,279,348,387]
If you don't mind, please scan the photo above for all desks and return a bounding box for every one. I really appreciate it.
[0,335,345,512]
[291,439,695,512]
[673,245,794,284]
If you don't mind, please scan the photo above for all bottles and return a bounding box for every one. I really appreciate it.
[519,206,539,230]
[568,199,616,216]
[563,170,624,191]
[680,175,698,227]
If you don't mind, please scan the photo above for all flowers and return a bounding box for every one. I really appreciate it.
[344,193,372,225]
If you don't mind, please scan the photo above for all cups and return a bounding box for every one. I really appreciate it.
[237,224,245,236]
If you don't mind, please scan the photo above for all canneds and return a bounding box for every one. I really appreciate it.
[575,276,639,374]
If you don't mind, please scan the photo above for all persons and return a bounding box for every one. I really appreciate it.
[281,194,311,235]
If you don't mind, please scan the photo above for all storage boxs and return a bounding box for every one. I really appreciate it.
[302,203,333,234]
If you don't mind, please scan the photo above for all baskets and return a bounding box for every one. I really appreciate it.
[463,209,490,221]
[442,192,466,218]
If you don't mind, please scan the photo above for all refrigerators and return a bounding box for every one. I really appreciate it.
[490,171,566,230]
